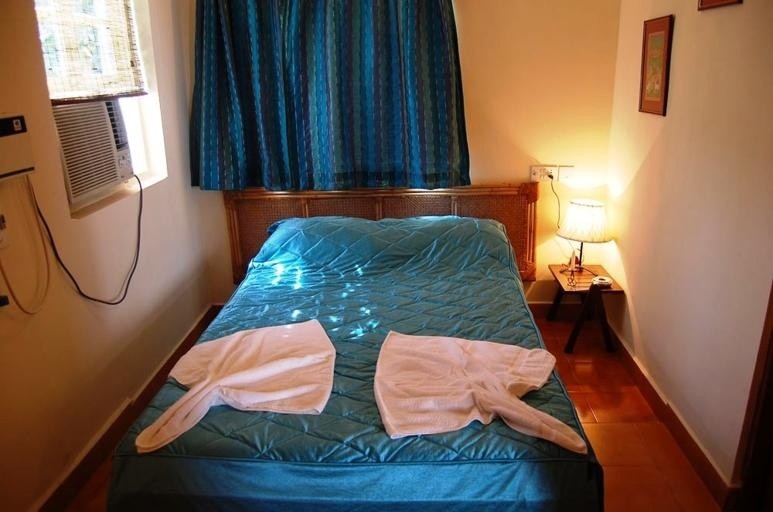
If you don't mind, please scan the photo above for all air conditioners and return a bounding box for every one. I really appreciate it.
[50,95,137,217]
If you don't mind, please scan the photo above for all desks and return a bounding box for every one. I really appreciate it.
[549,264,624,355]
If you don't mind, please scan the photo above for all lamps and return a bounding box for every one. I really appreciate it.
[555,198,616,276]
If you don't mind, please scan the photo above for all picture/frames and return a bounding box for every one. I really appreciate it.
[638,14,675,117]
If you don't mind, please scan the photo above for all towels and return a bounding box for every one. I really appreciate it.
[373,327,589,457]
[133,319,337,456]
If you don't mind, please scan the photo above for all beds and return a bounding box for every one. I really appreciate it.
[111,182,604,510]
